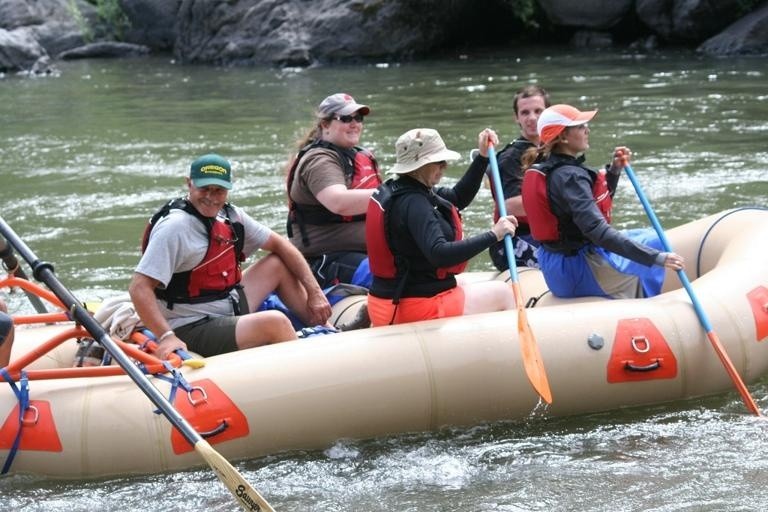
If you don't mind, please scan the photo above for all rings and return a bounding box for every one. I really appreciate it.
[675,260,679,265]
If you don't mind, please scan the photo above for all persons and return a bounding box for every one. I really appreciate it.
[285,93,384,289]
[484,85,552,272]
[366,127,520,328]
[520,104,687,300]
[0,234,15,371]
[128,155,343,361]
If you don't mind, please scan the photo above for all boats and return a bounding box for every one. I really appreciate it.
[0,207,767,483]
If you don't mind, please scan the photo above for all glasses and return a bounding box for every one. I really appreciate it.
[326,114,364,123]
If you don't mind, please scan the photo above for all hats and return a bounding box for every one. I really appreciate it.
[387,127,461,174]
[190,154,231,191]
[316,93,369,119]
[536,104,597,144]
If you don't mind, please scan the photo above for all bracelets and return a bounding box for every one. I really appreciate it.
[156,330,175,343]
[655,252,667,268]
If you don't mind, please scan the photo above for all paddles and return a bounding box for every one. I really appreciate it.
[0,213,276,512]
[616,150,763,416]
[487,140,554,406]
[82,301,204,369]
